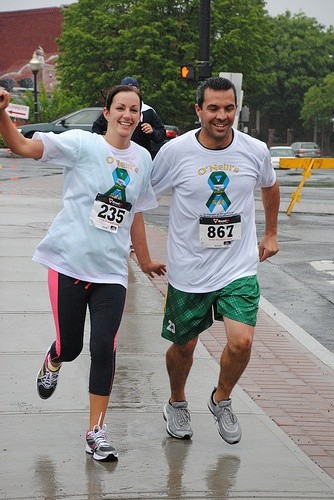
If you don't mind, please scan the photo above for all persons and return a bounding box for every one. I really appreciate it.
[0,85,168,461]
[92,77,166,161]
[152,77,280,445]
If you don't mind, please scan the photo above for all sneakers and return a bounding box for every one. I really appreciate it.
[36,344,62,400]
[207,386,242,445]
[85,411,119,462]
[163,398,194,440]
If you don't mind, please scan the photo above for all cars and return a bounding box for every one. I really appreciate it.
[17,107,179,158]
[291,141,322,158]
[269,145,297,169]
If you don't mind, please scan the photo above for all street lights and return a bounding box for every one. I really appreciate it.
[29,56,41,123]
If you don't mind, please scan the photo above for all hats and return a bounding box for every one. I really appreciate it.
[121,77,141,89]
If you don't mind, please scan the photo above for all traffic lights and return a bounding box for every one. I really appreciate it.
[180,64,194,80]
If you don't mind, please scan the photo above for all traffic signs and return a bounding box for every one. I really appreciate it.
[5,103,30,120]
